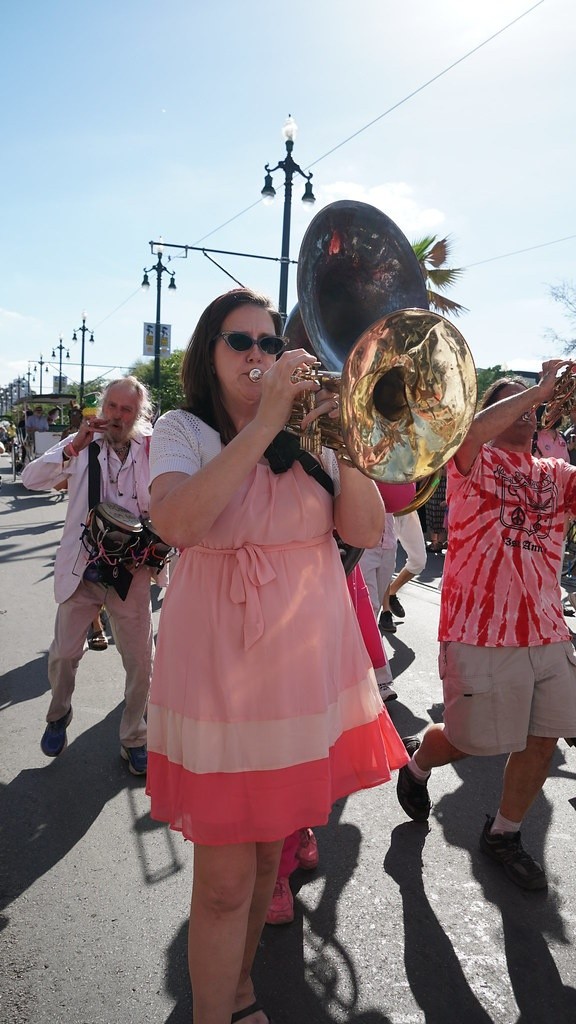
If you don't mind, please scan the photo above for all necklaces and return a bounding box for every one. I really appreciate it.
[112,442,131,456]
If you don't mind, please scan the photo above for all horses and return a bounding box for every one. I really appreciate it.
[60,399,86,441]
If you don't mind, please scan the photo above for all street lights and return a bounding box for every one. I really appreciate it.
[259,114,316,330]
[0,351,48,416]
[51,335,70,394]
[72,308,94,410]
[141,230,176,387]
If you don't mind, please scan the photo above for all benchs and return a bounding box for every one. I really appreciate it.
[24,425,72,436]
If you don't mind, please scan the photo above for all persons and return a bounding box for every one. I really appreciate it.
[21,376,180,776]
[0,406,61,454]
[260,480,428,924]
[59,409,84,442]
[396,359,576,892]
[146,288,386,1024]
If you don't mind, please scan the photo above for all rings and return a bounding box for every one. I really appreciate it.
[86,420,90,426]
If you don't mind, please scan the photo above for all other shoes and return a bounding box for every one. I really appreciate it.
[90,631,107,650]
[426,543,443,553]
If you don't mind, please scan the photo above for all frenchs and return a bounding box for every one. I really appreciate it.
[279,199,441,518]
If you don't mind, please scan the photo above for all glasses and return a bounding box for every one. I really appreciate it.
[219,331,287,356]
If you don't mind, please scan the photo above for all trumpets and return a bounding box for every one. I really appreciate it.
[248,307,479,486]
[521,357,576,431]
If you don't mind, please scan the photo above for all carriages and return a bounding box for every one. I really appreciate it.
[10,393,86,484]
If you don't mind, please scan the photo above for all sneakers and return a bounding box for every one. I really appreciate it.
[122,743,148,775]
[377,683,397,702]
[398,736,433,821]
[480,815,547,891]
[294,826,319,869]
[389,593,406,618]
[379,610,397,632]
[41,705,72,756]
[263,875,294,927]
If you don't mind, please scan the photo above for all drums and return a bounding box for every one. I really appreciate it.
[136,516,172,568]
[89,501,143,560]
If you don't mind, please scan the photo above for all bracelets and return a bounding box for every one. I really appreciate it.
[63,451,71,458]
[68,443,79,457]
